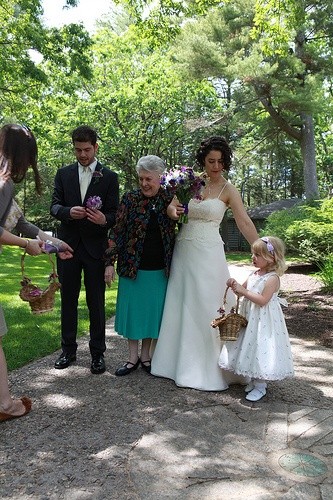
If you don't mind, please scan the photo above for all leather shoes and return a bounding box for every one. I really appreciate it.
[139,358,151,370]
[90,353,106,374]
[54,351,77,369]
[115,356,139,375]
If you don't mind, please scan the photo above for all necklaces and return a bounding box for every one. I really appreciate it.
[208,175,221,194]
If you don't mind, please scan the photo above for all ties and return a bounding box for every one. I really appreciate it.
[80,167,90,203]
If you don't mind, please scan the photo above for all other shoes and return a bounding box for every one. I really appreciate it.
[245,379,266,402]
[0,396,32,422]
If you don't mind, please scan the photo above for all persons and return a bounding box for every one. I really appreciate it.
[151,136,260,392]
[50,126,119,374]
[102,156,175,377]
[212,236,293,402]
[0,125,73,424]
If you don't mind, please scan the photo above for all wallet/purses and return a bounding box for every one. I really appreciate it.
[40,240,66,254]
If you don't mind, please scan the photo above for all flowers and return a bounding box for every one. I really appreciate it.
[86,196,102,210]
[159,164,207,224]
[29,289,42,296]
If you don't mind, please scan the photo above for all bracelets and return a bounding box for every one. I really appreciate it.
[20,239,29,249]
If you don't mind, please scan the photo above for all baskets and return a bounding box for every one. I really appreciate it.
[210,286,248,341]
[19,248,60,314]
